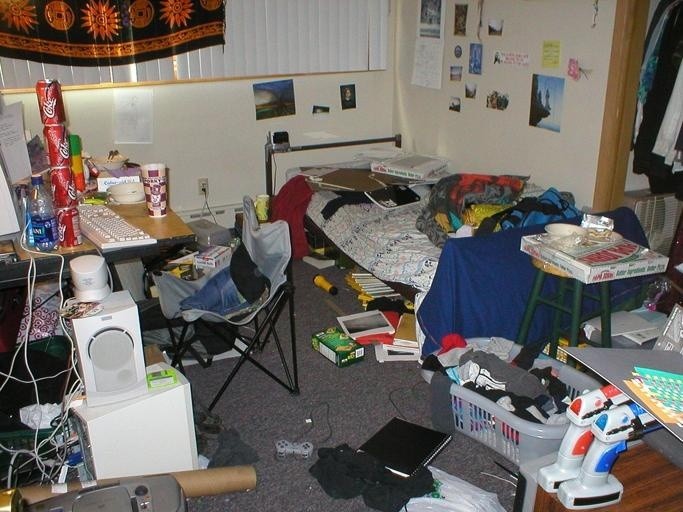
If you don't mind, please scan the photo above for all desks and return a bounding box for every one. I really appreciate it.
[518,440,682,512]
[1,200,193,303]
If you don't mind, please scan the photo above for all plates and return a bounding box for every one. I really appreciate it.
[587,231,615,244]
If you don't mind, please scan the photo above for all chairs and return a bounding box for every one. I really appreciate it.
[156,196,301,424]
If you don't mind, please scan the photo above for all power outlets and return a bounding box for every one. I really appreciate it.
[197,177,209,195]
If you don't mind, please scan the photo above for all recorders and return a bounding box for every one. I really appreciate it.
[24,475,187,512]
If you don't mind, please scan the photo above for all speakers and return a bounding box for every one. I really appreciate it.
[72,290,149,407]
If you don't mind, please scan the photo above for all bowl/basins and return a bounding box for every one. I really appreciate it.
[107,182,145,202]
[95,155,128,169]
[544,224,583,237]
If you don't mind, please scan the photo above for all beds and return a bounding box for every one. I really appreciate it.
[266,134,634,355]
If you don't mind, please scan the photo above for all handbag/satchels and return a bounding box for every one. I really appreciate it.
[148,237,270,322]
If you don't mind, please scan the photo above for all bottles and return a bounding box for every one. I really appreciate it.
[28,176,58,252]
[314,274,338,295]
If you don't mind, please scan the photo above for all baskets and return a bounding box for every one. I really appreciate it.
[420,337,602,468]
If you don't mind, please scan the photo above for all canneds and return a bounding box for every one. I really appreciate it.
[43,124,73,167]
[50,166,78,207]
[56,206,82,247]
[35,78,66,125]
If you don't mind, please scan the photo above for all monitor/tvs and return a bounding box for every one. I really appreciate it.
[0,154,22,241]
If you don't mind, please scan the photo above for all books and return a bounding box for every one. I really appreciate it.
[355,415,453,480]
[363,185,421,211]
[347,267,422,365]
[369,148,447,181]
[581,305,670,346]
[301,252,336,270]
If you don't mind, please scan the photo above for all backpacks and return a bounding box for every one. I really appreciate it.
[474,187,578,234]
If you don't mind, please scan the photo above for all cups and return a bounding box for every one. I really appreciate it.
[253,195,269,221]
[140,164,168,218]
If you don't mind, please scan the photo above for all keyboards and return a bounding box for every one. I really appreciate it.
[75,205,157,249]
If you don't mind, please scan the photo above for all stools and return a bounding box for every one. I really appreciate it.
[513,254,615,369]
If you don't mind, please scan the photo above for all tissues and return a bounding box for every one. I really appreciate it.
[97,168,140,193]
